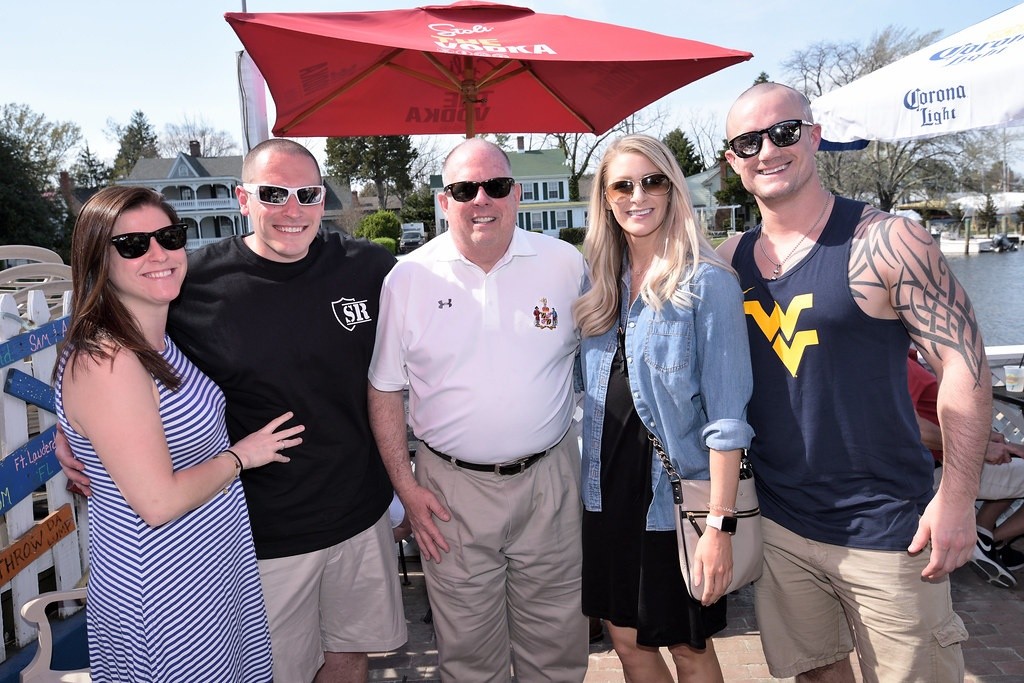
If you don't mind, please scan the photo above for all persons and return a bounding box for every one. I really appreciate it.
[571,133,756,683]
[716,83,993,683]
[367,137,589,683]
[389,494,413,543]
[54,140,398,683]
[50,186,306,683]
[905,349,1024,588]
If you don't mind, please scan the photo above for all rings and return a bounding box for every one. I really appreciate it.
[280,440,284,450]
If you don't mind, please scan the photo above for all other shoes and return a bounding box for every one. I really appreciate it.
[589,618,604,644]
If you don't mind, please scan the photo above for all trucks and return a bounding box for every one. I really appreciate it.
[397,222,429,255]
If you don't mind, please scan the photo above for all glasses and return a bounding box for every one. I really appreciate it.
[242,183,326,206]
[442,177,515,203]
[729,119,814,159]
[109,223,188,259]
[604,174,671,204]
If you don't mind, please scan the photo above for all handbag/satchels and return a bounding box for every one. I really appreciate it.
[671,468,764,601]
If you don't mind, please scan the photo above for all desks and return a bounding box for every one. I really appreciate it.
[924,363,1024,417]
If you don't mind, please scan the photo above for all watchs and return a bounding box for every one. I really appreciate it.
[706,514,737,535]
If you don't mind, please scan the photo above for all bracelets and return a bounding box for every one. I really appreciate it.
[223,449,243,477]
[214,455,236,494]
[709,505,737,513]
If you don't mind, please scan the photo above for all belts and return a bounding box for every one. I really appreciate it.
[421,425,571,477]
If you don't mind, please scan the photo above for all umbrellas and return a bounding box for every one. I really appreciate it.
[812,0,1024,150]
[224,0,754,139]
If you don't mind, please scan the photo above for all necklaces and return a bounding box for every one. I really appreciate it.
[760,191,831,274]
[632,266,643,274]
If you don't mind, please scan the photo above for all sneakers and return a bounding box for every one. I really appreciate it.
[994,540,1024,570]
[966,531,1019,592]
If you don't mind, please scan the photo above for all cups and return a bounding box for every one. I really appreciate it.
[1004,365,1024,392]
